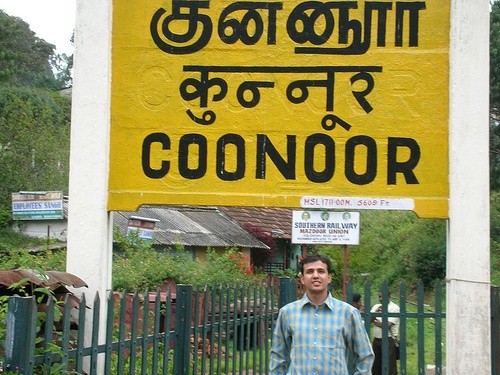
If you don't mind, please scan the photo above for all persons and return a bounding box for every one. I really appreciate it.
[349,289,401,375]
[269,255,375,375]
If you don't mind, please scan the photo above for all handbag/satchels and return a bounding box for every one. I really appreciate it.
[395,340,400,360]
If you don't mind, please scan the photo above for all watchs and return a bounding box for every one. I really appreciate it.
[374,319,377,323]
